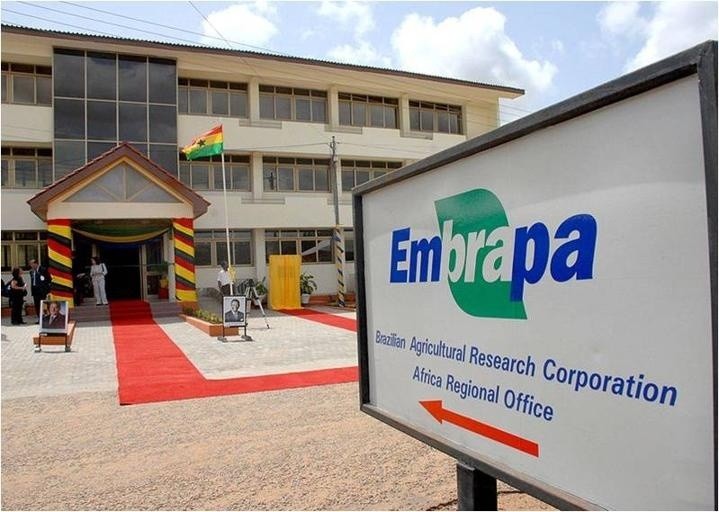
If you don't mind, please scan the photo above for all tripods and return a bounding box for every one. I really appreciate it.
[240,279,270,329]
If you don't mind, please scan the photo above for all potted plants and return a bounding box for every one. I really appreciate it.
[300,271,318,305]
[177,303,239,336]
[254,277,268,306]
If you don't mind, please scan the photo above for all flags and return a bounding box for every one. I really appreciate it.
[180,124,224,162]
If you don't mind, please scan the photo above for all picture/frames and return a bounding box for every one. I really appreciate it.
[39,300,69,335]
[223,296,247,328]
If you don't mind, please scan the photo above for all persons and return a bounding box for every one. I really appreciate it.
[29,258,52,325]
[71,248,87,306]
[40,301,65,329]
[216,259,236,306]
[89,255,110,306]
[223,297,245,322]
[8,266,30,325]
